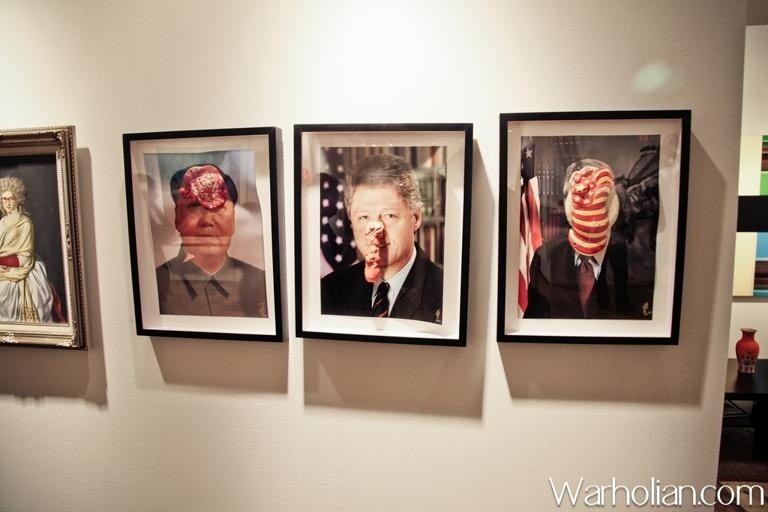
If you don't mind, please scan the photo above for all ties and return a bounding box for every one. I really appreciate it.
[372,281,390,317]
[576,255,595,319]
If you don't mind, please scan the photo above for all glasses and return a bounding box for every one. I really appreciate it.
[1,197,15,202]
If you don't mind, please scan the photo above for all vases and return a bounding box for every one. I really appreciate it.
[735,329,760,374]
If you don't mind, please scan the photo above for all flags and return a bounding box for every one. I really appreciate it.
[519,136,544,314]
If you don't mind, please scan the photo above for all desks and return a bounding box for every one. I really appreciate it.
[724,357,767,462]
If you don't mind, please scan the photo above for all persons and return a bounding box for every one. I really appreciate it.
[1,176,68,326]
[518,157,653,321]
[155,164,268,320]
[320,153,444,326]
